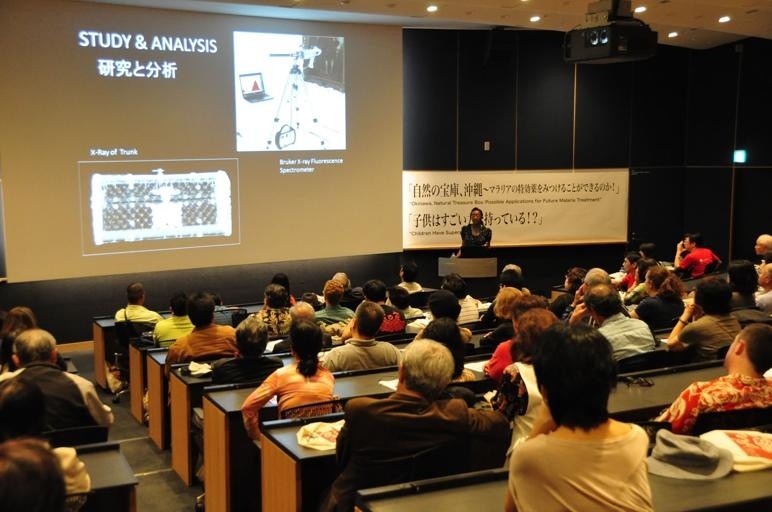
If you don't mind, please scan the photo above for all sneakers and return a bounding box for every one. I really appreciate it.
[112,386,129,403]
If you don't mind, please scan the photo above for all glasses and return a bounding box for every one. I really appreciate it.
[621,376,654,387]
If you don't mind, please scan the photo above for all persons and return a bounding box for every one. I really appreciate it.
[504,321,653,512]
[455,206,493,259]
[318,338,509,512]
[115,232,772,437]
[0,305,115,512]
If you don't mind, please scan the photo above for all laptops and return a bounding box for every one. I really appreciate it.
[239,72,274,103]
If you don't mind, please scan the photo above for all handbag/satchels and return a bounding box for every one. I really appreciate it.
[140,331,154,344]
[701,427,772,472]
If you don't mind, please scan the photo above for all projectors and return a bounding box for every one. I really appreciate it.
[562,21,658,65]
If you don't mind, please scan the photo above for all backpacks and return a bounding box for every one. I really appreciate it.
[733,309,772,326]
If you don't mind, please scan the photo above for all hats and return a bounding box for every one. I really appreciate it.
[180,364,213,377]
[302,292,323,308]
[503,263,521,277]
[647,428,733,480]
